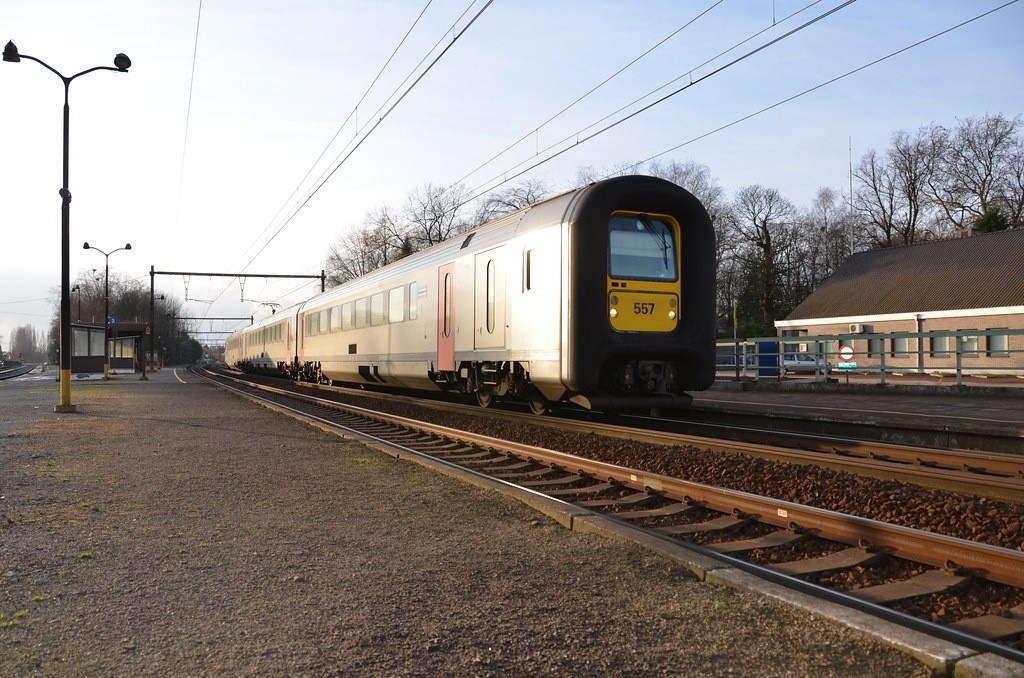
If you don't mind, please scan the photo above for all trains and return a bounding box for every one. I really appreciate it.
[224,174,718,409]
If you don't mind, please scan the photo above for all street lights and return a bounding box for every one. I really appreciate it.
[159,314,171,332]
[150,295,165,371]
[71,284,82,323]
[2,39,133,413]
[83,242,132,384]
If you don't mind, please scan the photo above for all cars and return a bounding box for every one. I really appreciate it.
[776,352,833,375]
